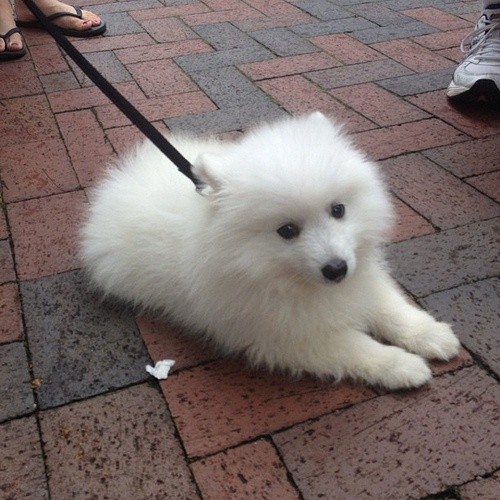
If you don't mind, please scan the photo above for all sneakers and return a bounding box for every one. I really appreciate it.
[446,3,500,105]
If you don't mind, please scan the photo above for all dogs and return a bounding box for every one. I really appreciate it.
[72,104,463,390]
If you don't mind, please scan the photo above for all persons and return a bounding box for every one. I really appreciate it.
[447,0,500,108]
[0,0,106,61]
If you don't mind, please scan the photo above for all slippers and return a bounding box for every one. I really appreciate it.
[14,5,106,37]
[0,27,27,59]
